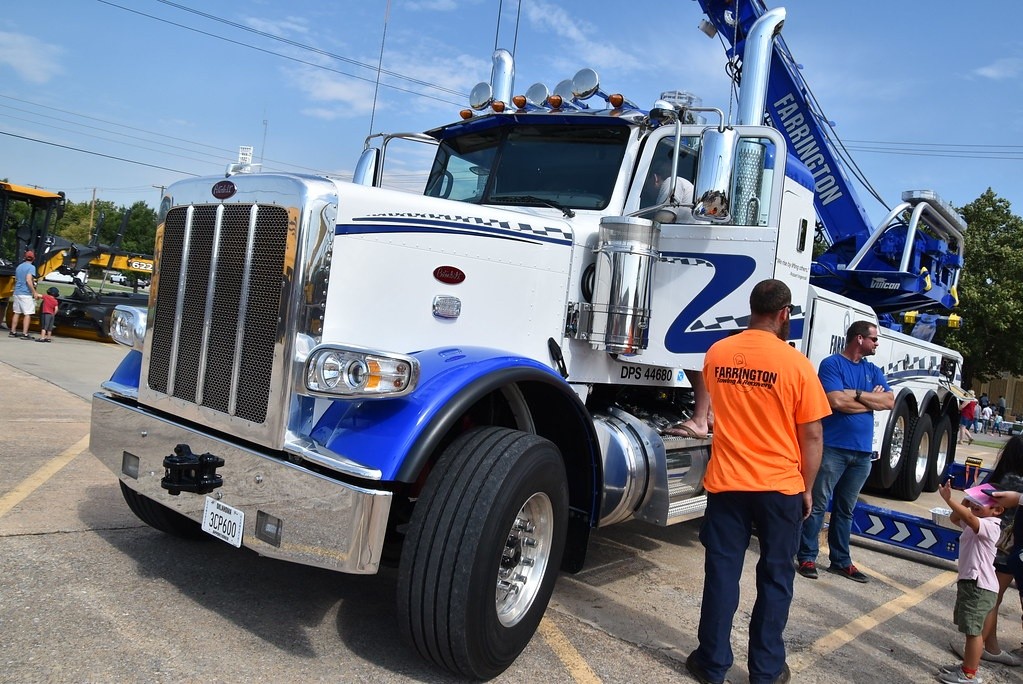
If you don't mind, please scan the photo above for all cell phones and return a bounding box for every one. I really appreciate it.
[981,489,1005,498]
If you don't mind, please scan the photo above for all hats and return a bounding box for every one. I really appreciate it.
[26,251,35,260]
[47,287,59,297]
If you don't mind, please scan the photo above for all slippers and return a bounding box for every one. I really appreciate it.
[662,424,713,439]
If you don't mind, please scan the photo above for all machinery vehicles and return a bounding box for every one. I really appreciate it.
[87,0,994,684]
[0,182,153,342]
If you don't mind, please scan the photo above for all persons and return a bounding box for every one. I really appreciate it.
[958,390,1007,446]
[939,435,1023,684]
[643,150,714,440]
[0,250,60,342]
[795,321,895,583]
[686,276,832,684]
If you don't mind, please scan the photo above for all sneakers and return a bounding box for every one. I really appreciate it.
[686,649,726,684]
[940,664,979,684]
[8,331,22,337]
[950,641,966,659]
[20,334,36,340]
[828,564,869,583]
[798,562,819,578]
[981,649,1022,667]
[773,662,791,684]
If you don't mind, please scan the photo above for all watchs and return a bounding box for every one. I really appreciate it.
[854,388,862,401]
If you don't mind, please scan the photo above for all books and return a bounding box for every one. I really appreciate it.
[963,483,999,507]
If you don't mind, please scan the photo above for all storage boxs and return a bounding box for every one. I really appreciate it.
[1013,424,1023,432]
[1002,421,1014,431]
[1005,415,1017,423]
[928,507,964,532]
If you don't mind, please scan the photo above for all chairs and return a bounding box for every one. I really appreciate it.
[533,159,616,198]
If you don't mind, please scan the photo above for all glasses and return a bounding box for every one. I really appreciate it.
[862,335,879,342]
[779,304,794,313]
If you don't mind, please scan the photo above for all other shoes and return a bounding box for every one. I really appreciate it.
[0,325,8,330]
[957,440,963,446]
[34,338,51,342]
[968,437,974,445]
[1007,646,1023,658]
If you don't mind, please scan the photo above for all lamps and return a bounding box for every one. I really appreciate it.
[697,17,719,38]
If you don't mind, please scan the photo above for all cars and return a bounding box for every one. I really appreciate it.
[110,274,145,289]
[42,268,88,287]
